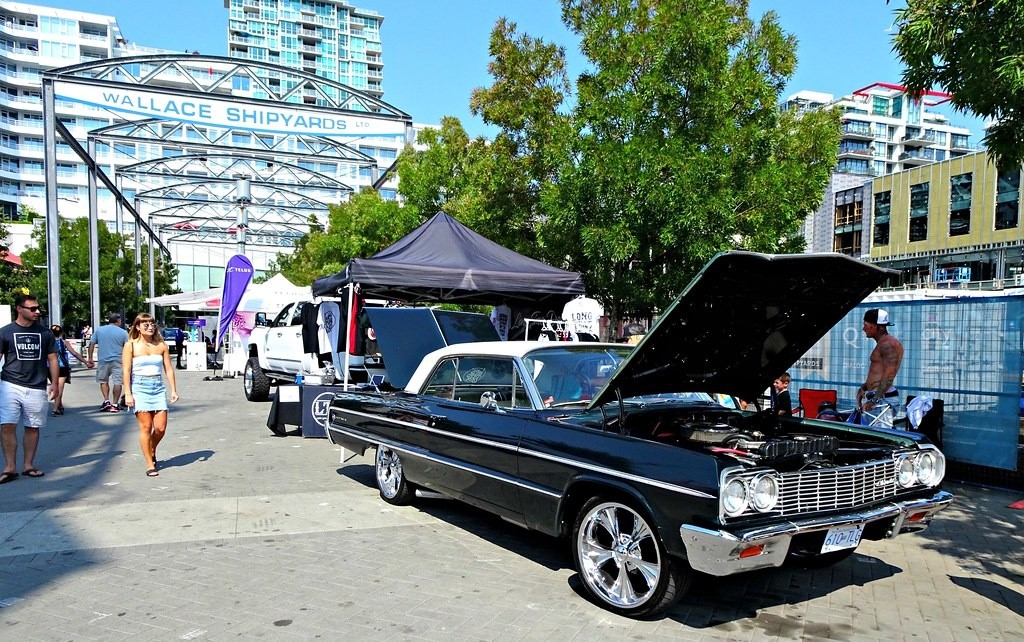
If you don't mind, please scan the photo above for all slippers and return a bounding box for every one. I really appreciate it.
[151,453,157,466]
[22,468,44,477]
[146,469,159,476]
[0,472,19,484]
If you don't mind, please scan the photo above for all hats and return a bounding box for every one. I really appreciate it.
[51,325,62,332]
[864,309,895,326]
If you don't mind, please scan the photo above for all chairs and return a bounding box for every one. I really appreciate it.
[207,341,223,370]
[791,389,837,420]
[893,395,946,452]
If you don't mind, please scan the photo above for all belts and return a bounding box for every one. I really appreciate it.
[867,390,898,400]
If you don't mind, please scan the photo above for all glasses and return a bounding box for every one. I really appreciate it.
[20,306,40,312]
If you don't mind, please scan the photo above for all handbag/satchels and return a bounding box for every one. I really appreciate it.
[65,367,71,384]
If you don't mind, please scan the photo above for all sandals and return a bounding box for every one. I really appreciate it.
[52,410,64,416]
[58,407,65,412]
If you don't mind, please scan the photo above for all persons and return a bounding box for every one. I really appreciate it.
[712,393,748,411]
[122,313,179,477]
[857,308,904,428]
[773,372,793,417]
[0,295,60,484]
[48,313,217,417]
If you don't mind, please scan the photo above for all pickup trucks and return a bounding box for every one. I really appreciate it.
[242,298,402,404]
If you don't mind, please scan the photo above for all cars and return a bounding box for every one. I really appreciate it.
[322,247,955,620]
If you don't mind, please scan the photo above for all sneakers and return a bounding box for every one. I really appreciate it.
[99,401,111,411]
[110,403,119,412]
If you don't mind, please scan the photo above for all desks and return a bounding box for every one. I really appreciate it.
[266,384,377,439]
[186,342,206,371]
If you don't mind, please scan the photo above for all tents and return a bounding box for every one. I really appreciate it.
[180,273,311,377]
[312,209,588,465]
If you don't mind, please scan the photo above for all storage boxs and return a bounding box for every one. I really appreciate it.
[304,375,336,386]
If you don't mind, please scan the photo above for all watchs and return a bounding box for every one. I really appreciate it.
[872,397,879,403]
